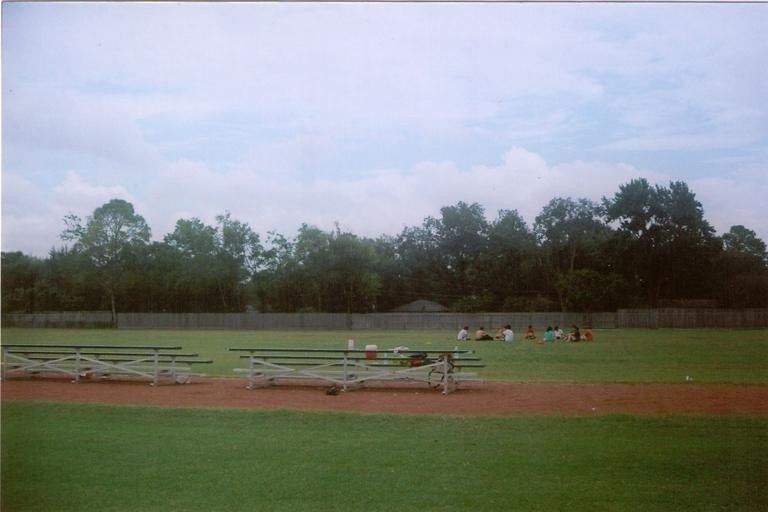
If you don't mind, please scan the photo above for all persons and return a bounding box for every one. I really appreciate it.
[455,322,593,345]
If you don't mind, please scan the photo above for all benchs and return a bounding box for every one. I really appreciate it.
[226,345,486,396]
[1,343,214,389]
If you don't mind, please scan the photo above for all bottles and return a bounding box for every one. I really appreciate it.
[454,346,458,358]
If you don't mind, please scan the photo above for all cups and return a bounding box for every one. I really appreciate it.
[348,340,353,350]
